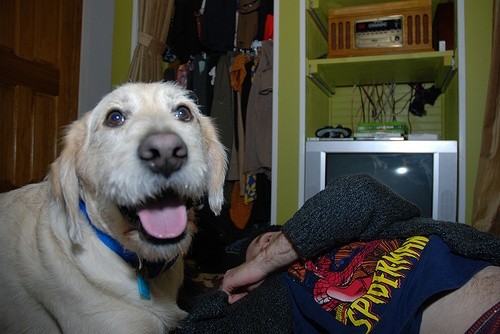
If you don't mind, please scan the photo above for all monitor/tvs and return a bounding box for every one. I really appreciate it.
[304,139,457,224]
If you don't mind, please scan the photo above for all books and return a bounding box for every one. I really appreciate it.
[354,122,408,140]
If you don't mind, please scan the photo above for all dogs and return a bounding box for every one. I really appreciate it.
[0,80,230,334]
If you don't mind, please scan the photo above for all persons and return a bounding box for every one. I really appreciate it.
[169,174,500,334]
